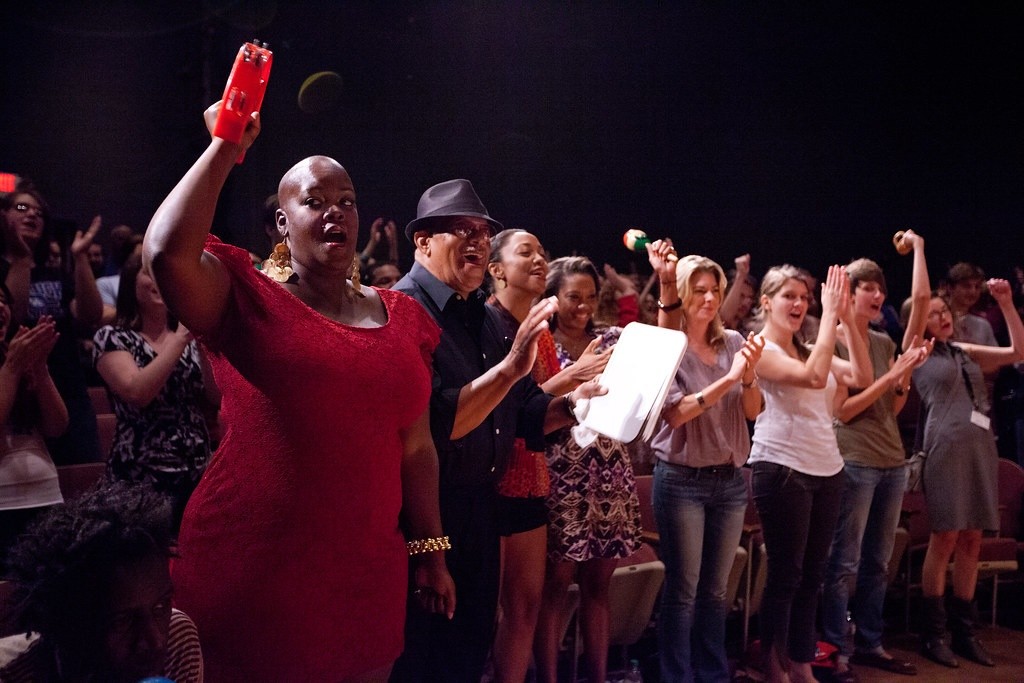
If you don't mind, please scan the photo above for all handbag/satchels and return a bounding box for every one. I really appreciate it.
[904,451,927,494]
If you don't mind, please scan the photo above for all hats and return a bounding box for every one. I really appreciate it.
[405,179,505,249]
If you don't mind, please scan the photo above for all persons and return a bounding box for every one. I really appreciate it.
[142,95,454,683]
[0,176,1024,683]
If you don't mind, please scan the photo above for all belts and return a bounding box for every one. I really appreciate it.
[688,464,733,475]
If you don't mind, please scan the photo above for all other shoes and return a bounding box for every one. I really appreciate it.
[852,649,917,674]
[827,663,858,682]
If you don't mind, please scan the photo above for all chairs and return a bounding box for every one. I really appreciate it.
[558,457,1024,683]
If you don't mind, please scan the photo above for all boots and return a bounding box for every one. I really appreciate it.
[919,593,996,668]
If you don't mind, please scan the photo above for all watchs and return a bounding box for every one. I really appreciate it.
[893,385,910,396]
[741,377,757,389]
[562,393,576,419]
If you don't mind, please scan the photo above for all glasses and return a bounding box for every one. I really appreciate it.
[448,221,497,243]
[13,202,47,216]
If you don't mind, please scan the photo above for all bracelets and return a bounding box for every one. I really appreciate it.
[657,298,683,312]
[407,536,451,556]
[695,392,711,410]
